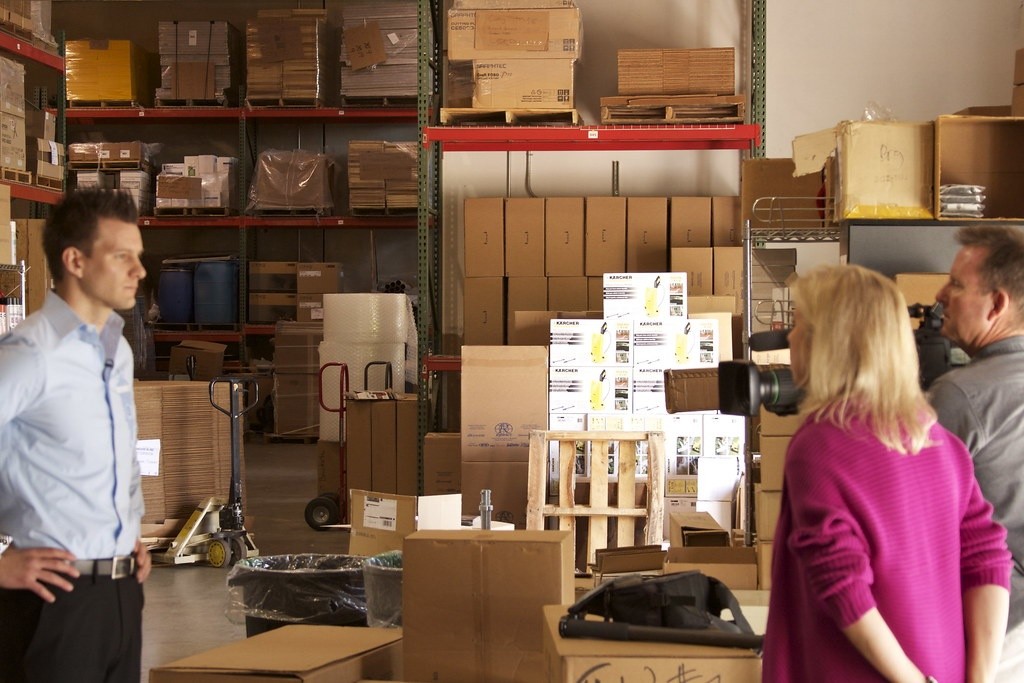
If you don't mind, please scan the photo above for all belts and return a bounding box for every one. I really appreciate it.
[60,556,139,580]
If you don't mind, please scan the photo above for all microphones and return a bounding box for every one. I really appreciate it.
[748,329,795,351]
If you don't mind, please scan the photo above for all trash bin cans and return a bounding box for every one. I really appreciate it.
[226,553,364,639]
[364,550,403,628]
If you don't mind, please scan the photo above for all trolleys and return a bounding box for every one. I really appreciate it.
[304,361,394,533]
[149,376,260,570]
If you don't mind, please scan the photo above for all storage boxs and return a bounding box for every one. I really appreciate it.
[0,1,1024,683]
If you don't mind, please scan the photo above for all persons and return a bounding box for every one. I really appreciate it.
[926,224,1023,683]
[761,262,1014,683]
[0,181,152,683]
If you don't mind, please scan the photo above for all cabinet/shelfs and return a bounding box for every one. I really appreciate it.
[63,105,431,439]
[0,30,70,341]
[415,1,766,493]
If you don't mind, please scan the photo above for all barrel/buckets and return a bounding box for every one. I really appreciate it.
[229,550,405,638]
[157,261,236,323]
